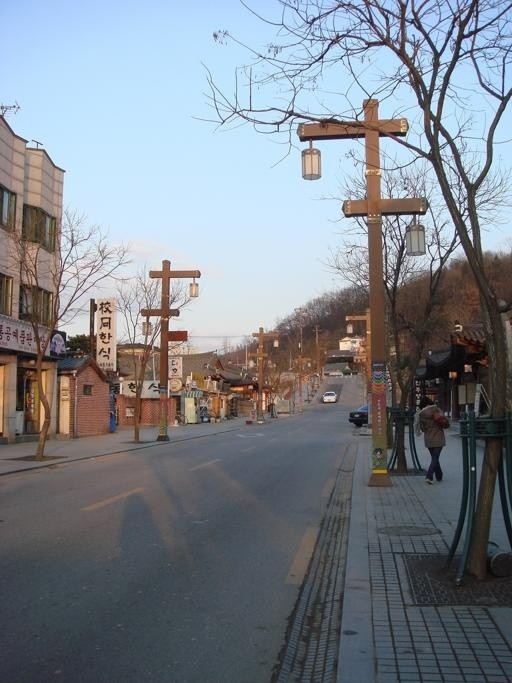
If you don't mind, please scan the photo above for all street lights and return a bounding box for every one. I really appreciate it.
[344,307,371,382]
[250,326,280,424]
[297,98,429,489]
[141,258,200,440]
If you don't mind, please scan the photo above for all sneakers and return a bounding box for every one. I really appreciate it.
[436,477,441,481]
[425,478,433,484]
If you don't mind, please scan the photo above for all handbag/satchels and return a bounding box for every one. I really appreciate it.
[434,412,449,428]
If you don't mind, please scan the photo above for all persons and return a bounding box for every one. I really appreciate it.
[50,334,66,356]
[415,396,445,484]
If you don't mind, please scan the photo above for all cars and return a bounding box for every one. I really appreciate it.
[348,404,390,426]
[323,391,337,402]
